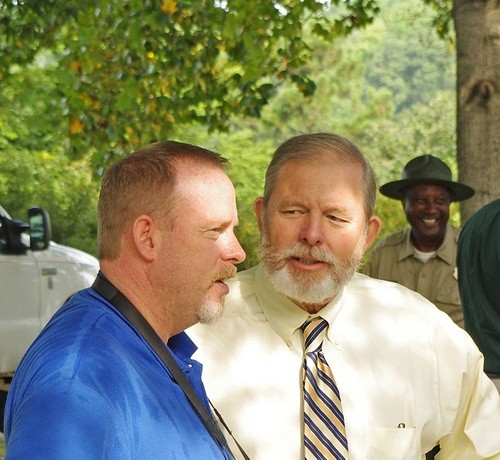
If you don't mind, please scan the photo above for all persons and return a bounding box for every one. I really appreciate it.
[364,155,476,331]
[456,198,500,397]
[179,133,500,460]
[4,140,246,460]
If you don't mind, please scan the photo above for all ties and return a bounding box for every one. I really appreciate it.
[299,318,349,460]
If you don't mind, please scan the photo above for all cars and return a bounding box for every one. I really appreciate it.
[1,201,100,376]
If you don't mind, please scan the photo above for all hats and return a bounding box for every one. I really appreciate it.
[379,155,475,201]
